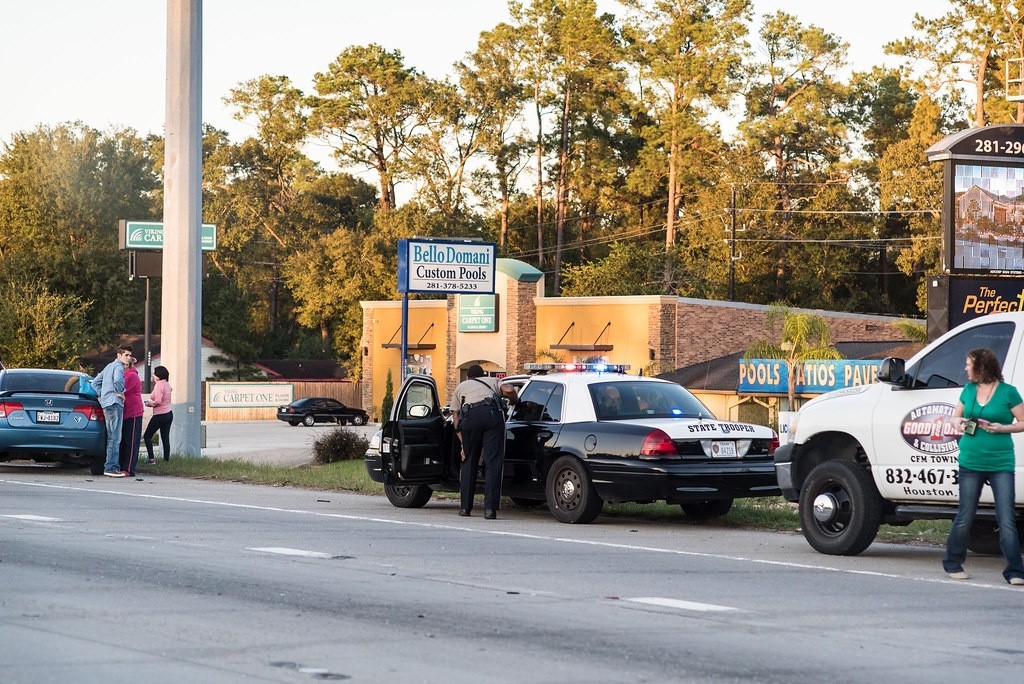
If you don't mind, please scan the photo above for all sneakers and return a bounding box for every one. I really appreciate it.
[103,469,126,477]
[143,459,156,465]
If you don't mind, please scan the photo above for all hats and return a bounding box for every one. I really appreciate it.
[467,365,484,377]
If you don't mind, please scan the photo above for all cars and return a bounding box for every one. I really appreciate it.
[277,397,371,426]
[362,364,780,524]
[1,366,108,464]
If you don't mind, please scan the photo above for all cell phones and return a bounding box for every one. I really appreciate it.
[978,418,991,427]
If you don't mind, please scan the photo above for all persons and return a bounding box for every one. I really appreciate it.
[91,345,133,477]
[943,348,1024,585]
[118,356,144,477]
[143,366,173,465]
[449,365,519,520]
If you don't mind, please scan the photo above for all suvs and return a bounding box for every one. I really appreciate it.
[772,310,1024,560]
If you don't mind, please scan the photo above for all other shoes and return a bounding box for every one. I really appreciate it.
[458,508,470,516]
[1009,577,1024,585]
[122,470,135,476]
[483,508,497,519]
[944,571,970,579]
